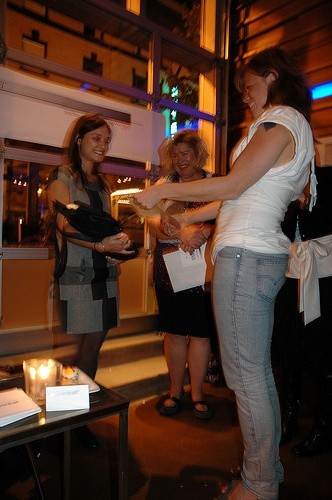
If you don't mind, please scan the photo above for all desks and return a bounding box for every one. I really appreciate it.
[0,374,132,500]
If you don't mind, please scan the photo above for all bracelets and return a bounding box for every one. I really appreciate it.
[102,242,106,254]
[94,243,97,251]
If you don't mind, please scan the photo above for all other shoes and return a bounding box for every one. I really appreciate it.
[189,391,212,419]
[159,390,187,416]
[202,480,260,500]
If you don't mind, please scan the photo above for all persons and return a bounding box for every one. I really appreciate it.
[125,47,316,500]
[132,130,219,414]
[44,113,136,379]
